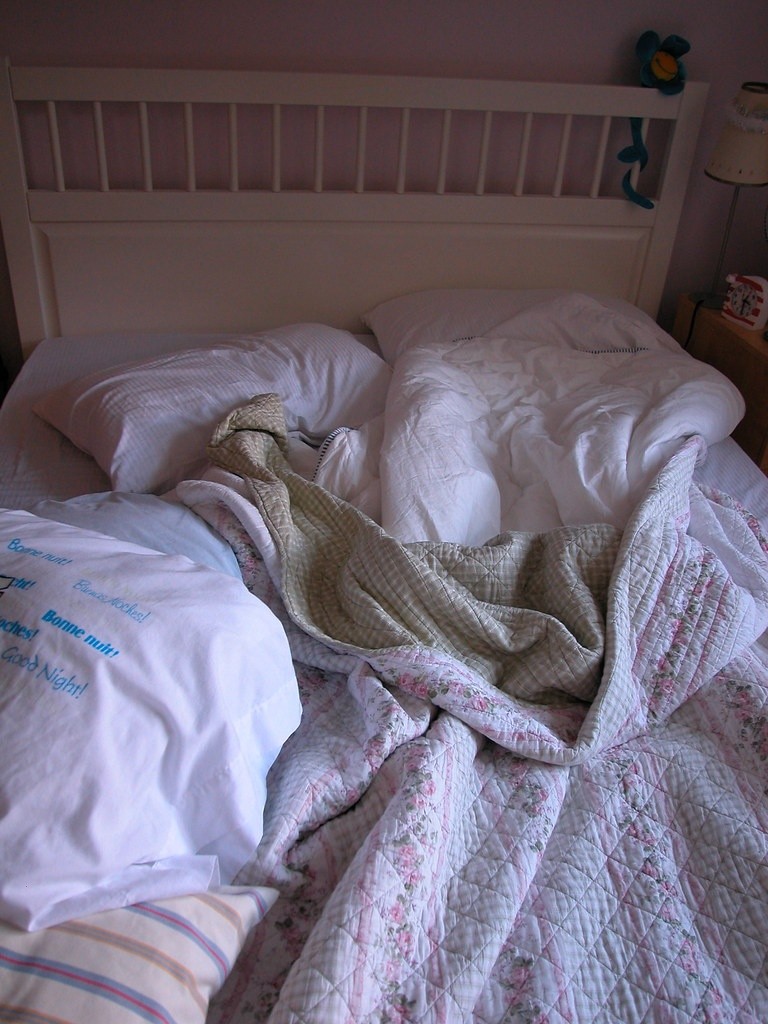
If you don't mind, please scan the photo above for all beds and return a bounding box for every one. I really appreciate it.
[0,52,768,1024]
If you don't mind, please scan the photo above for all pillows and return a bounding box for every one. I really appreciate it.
[358,288,569,369]
[0,507,307,934]
[32,321,394,496]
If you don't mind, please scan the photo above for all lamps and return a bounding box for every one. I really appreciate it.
[688,82,767,309]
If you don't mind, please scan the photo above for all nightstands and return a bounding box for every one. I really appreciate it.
[672,290,768,480]
